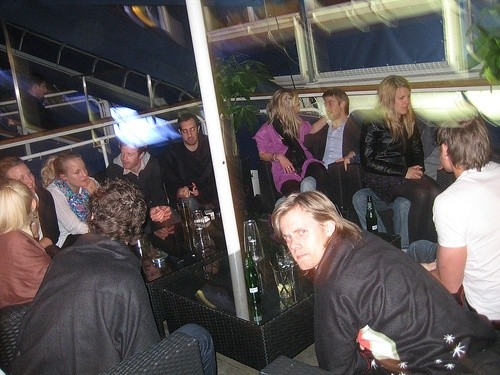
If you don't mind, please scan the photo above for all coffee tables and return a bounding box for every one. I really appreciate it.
[161,223,402,371]
[128,208,227,340]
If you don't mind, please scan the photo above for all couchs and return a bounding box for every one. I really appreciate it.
[251,109,456,233]
[0,293,205,375]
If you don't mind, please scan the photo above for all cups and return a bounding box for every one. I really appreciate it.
[272,249,296,298]
[243,220,264,262]
[188,210,210,254]
[178,208,192,226]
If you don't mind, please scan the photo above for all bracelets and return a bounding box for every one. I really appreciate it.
[271,154,276,162]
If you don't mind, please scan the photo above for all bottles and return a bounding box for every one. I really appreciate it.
[244,252,265,304]
[366,196,378,235]
[179,200,188,207]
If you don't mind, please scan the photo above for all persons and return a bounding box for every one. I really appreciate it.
[11,179,217,375]
[270,192,499,375]
[0,74,500,329]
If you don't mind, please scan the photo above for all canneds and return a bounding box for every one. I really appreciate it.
[135,239,145,249]
[152,255,167,270]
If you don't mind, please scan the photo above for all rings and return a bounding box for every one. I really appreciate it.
[287,166,290,168]
